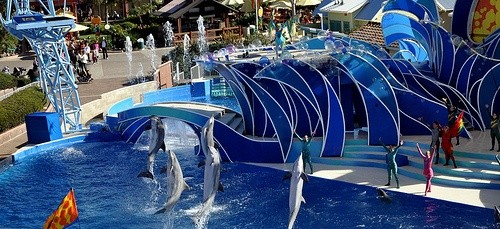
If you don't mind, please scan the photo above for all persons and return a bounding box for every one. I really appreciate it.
[441,98,462,145]
[379,137,403,189]
[416,143,436,196]
[418,118,451,165]
[270,15,292,63]
[294,128,316,174]
[487,105,500,152]
[33,36,108,81]
[439,120,460,168]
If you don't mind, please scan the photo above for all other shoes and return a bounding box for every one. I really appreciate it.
[385,183,390,186]
[396,186,400,189]
[490,148,494,151]
[434,159,438,164]
[454,164,457,168]
[443,163,449,166]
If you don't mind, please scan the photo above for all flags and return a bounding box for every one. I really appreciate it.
[43,190,78,229]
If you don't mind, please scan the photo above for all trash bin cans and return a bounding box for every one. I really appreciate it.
[138,39,145,49]
[250,27,256,35]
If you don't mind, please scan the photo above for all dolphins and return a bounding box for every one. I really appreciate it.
[152,149,191,218]
[196,113,225,160]
[282,151,310,229]
[136,114,167,181]
[377,186,394,203]
[189,144,225,229]
